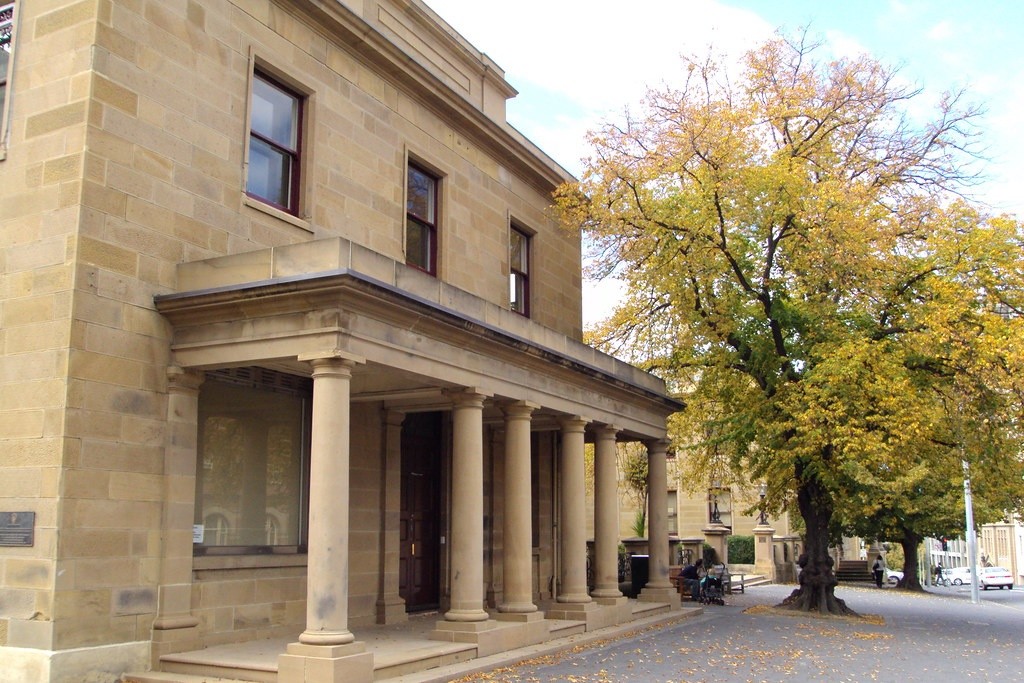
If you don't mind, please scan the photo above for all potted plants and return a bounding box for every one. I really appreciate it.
[703,543,716,567]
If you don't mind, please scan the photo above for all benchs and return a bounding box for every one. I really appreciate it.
[669,565,704,600]
[711,563,747,595]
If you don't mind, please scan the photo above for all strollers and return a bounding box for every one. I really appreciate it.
[696,561,726,606]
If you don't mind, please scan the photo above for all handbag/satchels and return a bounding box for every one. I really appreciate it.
[873,561,880,570]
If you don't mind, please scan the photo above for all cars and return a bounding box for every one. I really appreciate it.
[884,567,904,586]
[932,567,972,586]
[979,567,1014,591]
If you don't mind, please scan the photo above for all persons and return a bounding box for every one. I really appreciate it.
[678,558,704,601]
[872,555,885,588]
[701,569,717,587]
[934,562,947,587]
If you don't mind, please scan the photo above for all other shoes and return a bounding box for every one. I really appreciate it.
[692,597,700,600]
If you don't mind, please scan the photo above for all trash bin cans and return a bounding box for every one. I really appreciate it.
[630,555,649,596]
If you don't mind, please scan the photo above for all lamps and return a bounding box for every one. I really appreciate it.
[758,478,771,524]
[710,469,724,523]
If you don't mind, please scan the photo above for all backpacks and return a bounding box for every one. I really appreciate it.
[935,567,939,574]
